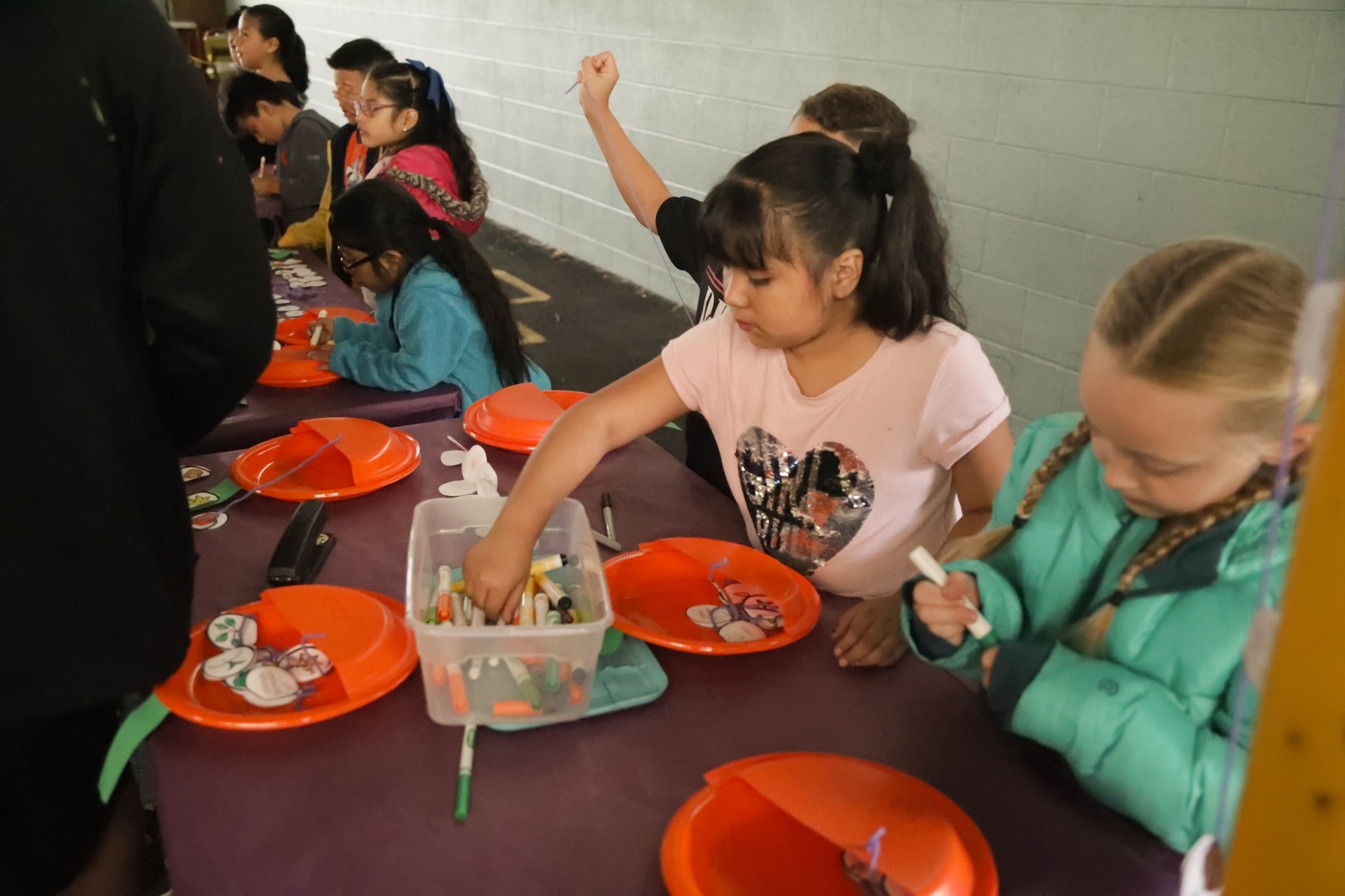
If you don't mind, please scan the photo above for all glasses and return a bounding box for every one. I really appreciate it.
[335,244,382,277]
[353,100,399,118]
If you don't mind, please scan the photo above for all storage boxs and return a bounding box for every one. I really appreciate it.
[404,497,614,728]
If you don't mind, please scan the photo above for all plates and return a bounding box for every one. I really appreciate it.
[462,382,593,455]
[659,751,998,896]
[601,537,821,656]
[276,306,376,345]
[256,345,340,388]
[229,418,420,502]
[153,584,419,730]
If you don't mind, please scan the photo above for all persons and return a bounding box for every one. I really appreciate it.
[1,0,279,894]
[460,133,1012,668]
[897,238,1325,862]
[210,4,915,492]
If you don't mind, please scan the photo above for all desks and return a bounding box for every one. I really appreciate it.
[150,418,1187,896]
[184,188,461,456]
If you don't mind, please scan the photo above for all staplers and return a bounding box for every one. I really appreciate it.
[266,499,336,588]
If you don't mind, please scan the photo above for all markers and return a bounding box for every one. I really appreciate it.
[310,309,327,350]
[258,156,266,178]
[601,493,616,541]
[593,530,621,551]
[908,546,1000,650]
[454,724,478,820]
[428,552,590,715]
